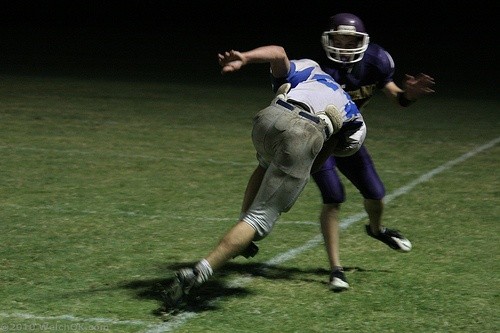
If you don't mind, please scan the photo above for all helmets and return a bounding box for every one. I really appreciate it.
[326,13,365,45]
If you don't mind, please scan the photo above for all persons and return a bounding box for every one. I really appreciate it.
[302,12,435,292]
[170,45,367,307]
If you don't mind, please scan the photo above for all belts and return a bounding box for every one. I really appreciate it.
[276,99,329,140]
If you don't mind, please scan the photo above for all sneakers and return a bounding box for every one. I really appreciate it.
[329,264,350,290]
[364,223,412,252]
[162,262,197,317]
